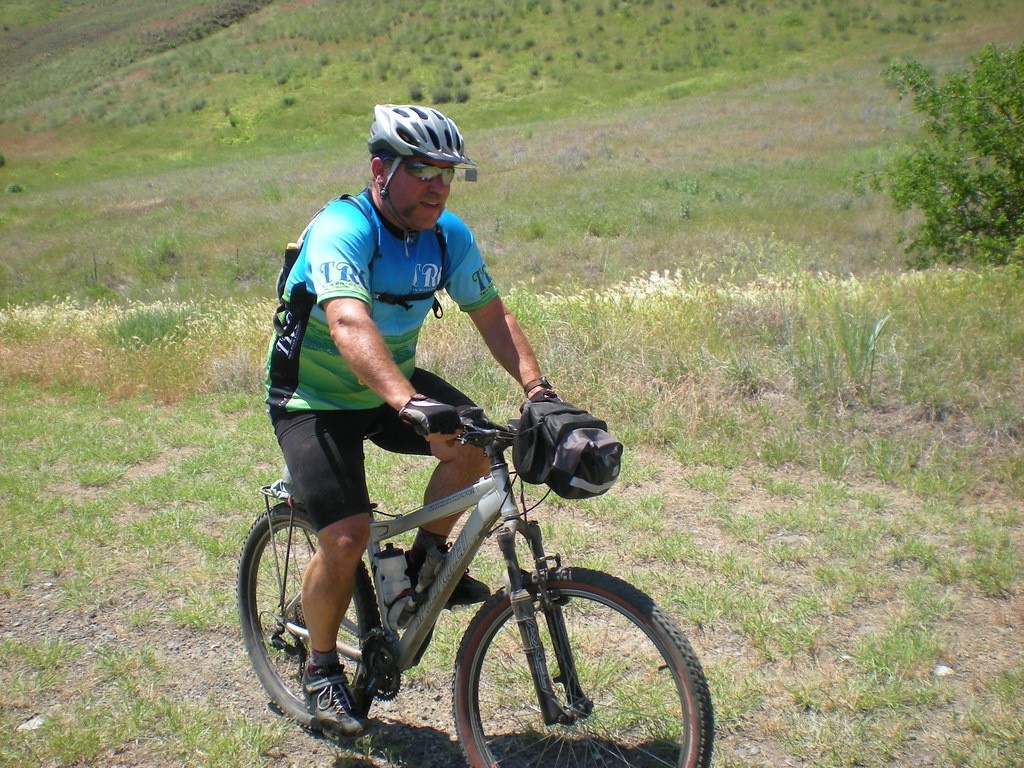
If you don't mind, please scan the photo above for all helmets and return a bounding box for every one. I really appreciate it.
[367,105,476,166]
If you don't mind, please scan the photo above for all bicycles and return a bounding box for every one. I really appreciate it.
[235,406,715,768]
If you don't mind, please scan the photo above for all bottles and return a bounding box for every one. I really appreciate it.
[372,542,411,631]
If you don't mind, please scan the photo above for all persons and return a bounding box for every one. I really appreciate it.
[264,103,559,737]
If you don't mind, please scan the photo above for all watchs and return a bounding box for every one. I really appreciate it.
[523,375,554,398]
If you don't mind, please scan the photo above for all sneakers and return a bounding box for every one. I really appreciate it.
[404,542,491,604]
[300,662,370,741]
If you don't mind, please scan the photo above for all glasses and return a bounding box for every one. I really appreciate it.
[382,156,455,186]
